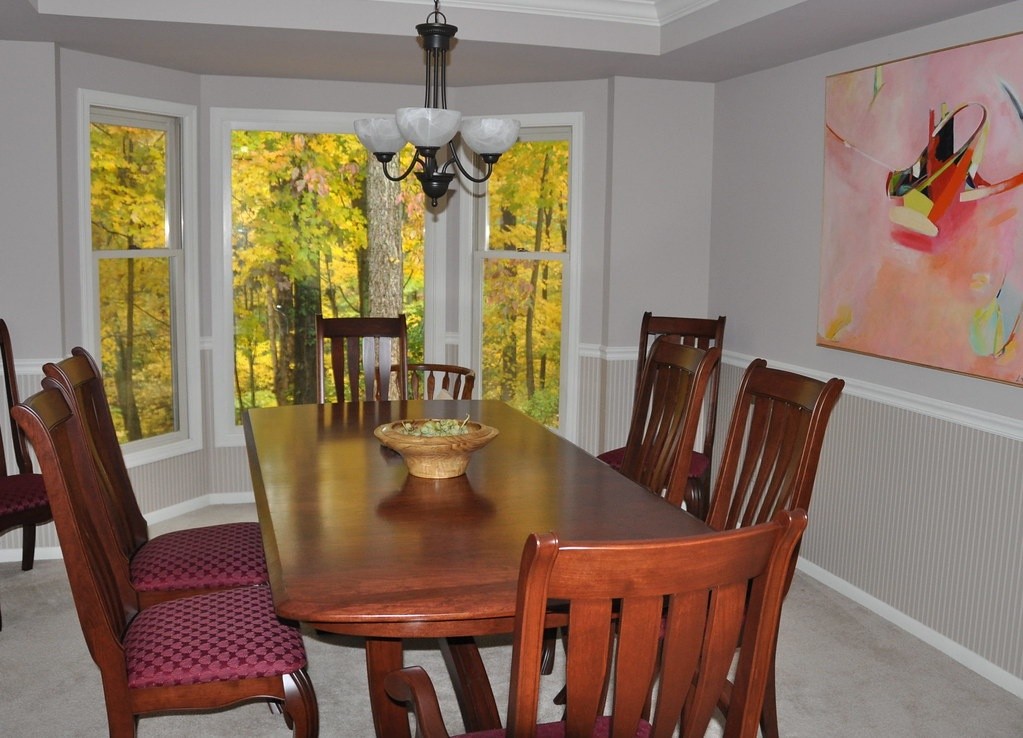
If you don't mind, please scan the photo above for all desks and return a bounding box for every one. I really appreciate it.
[243,397,720,732]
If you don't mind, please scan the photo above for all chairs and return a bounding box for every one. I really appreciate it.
[595,311,726,519]
[40,343,271,608]
[314,312,407,401]
[373,362,476,399]
[551,359,844,738]
[0,316,53,629]
[387,509,808,738]
[11,387,319,738]
[540,338,722,678]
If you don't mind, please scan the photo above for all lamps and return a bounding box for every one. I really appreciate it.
[351,0,521,208]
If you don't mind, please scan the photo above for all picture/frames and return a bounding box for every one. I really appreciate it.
[815,32,1023,386]
